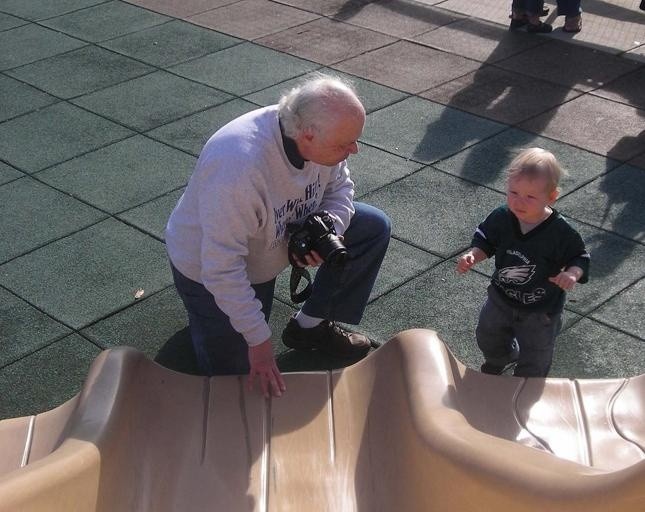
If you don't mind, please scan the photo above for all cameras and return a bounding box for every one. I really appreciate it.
[288,209,349,267]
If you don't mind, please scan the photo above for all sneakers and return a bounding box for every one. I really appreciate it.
[528,22,553,33]
[282,312,371,358]
[510,18,528,27]
[565,10,582,32]
[481,359,518,375]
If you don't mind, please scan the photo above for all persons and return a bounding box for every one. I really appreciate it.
[458,148,592,374]
[165,72,393,399]
[509,0,553,33]
[540,0,583,32]
